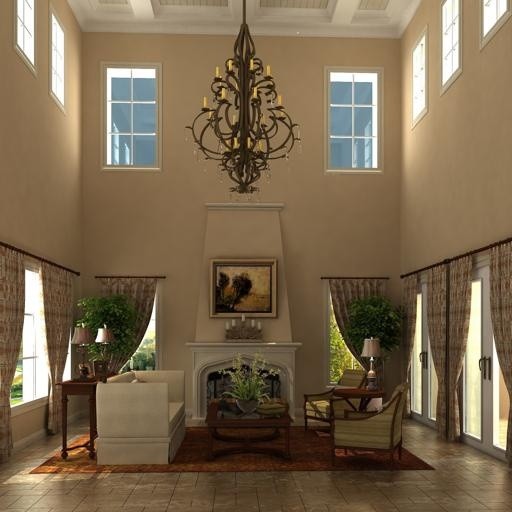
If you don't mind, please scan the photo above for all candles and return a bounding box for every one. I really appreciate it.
[226,312,262,330]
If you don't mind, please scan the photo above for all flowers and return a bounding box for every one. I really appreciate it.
[219,351,280,403]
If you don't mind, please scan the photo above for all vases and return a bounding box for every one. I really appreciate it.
[236,397,260,415]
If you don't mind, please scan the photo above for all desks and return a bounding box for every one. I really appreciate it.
[56,372,116,460]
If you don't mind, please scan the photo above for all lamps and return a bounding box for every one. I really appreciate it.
[186,1,301,195]
[361,338,383,390]
[68,322,116,382]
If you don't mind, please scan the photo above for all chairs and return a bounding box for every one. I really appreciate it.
[94,369,187,464]
[304,368,409,462]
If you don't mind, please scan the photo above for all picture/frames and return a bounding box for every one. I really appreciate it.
[209,258,277,318]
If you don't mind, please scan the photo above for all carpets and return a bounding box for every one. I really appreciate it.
[30,426,433,473]
